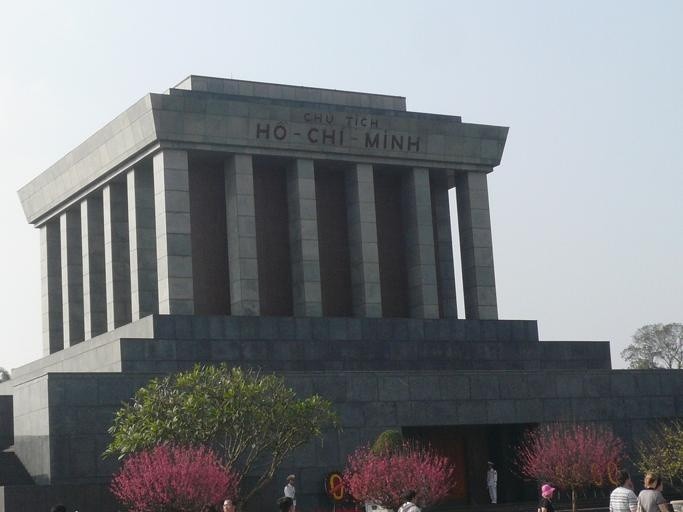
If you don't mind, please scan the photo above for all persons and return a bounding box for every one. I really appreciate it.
[221,496,235,512]
[276,497,294,512]
[607,470,670,512]
[485,461,497,505]
[536,484,555,511]
[397,490,419,512]
[283,474,296,510]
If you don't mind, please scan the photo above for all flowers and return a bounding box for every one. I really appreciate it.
[323,471,348,502]
[554,461,622,486]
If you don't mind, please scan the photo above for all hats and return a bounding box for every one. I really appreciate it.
[285,473,295,481]
[487,461,495,466]
[540,484,555,496]
